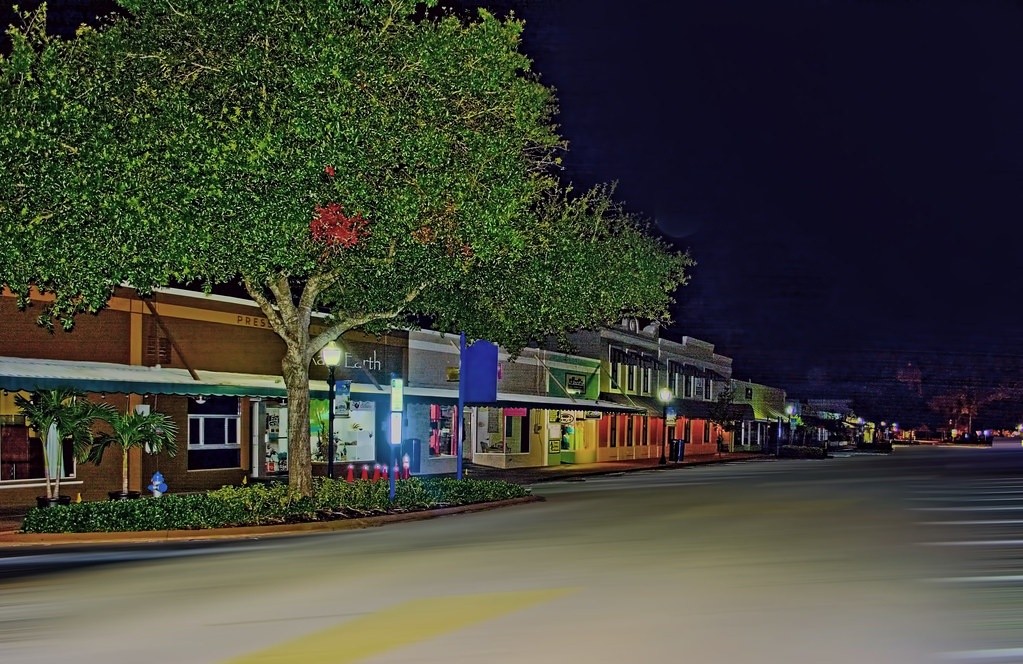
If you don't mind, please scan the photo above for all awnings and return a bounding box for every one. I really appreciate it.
[669,361,727,381]
[600,393,754,421]
[611,347,668,370]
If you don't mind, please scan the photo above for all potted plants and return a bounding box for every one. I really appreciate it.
[13,386,114,509]
[89,405,179,501]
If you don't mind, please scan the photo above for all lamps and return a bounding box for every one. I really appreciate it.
[196,395,205,404]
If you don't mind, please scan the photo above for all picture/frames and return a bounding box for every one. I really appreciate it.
[565,372,586,394]
